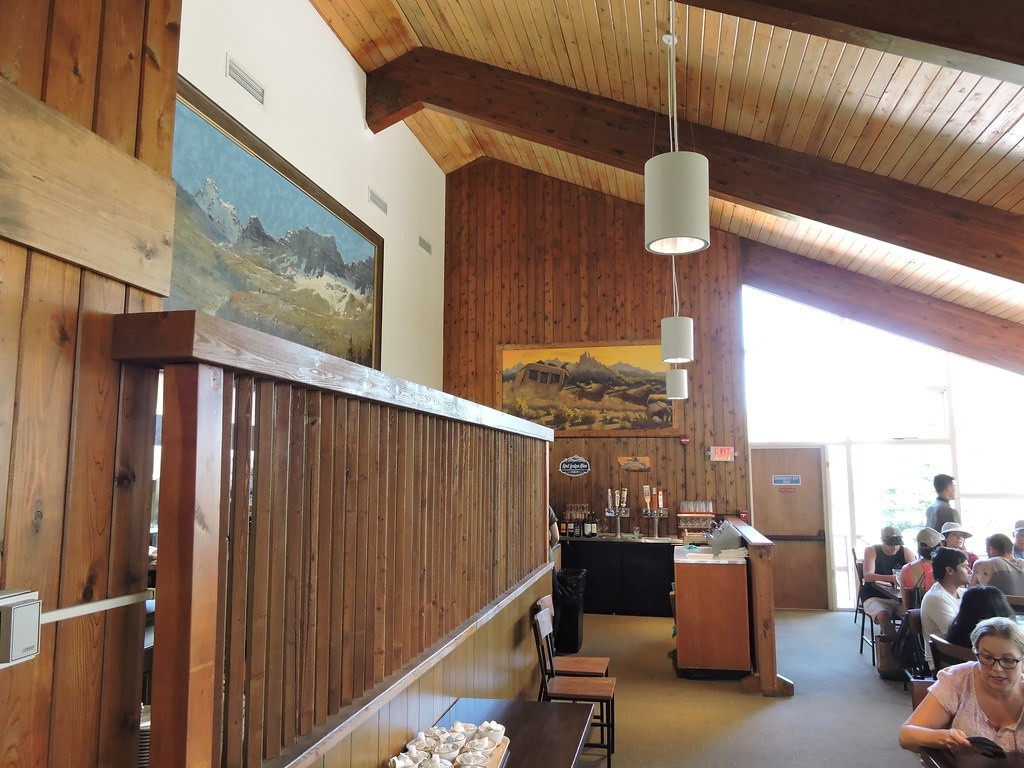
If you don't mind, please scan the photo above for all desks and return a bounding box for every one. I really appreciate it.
[430,698,594,768]
[918,746,1024,768]
[672,544,751,678]
[871,581,903,602]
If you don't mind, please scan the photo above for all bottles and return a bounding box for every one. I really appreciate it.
[559,511,597,538]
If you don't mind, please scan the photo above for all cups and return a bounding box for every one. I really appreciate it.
[892,569,902,589]
[633,527,639,538]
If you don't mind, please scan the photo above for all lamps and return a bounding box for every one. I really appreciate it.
[661,255,694,365]
[666,364,688,399]
[644,0,711,255]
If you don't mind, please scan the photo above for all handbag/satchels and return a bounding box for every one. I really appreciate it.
[874,635,904,678]
[891,618,921,667]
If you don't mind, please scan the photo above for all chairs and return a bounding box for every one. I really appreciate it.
[530,608,617,768]
[854,563,1024,729]
[531,594,610,743]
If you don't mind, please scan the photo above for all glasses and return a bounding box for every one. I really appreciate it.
[974,647,1024,669]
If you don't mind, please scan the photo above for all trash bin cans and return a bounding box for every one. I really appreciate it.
[553,567,587,657]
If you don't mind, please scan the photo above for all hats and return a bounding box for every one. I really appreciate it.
[881,526,904,545]
[1014,520,1024,532]
[917,527,945,549]
[941,522,973,538]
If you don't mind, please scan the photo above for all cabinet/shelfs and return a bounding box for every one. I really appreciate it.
[562,541,674,616]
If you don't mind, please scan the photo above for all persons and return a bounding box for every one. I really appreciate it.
[920,548,974,680]
[900,528,945,611]
[942,521,979,569]
[549,507,560,563]
[926,474,962,532]
[899,617,1024,753]
[944,586,1017,647]
[971,533,1024,597]
[1012,520,1024,560]
[861,525,916,630]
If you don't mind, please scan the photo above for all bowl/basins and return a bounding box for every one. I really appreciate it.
[389,724,505,768]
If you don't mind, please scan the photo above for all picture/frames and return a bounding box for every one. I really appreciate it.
[495,340,684,437]
[164,72,384,372]
